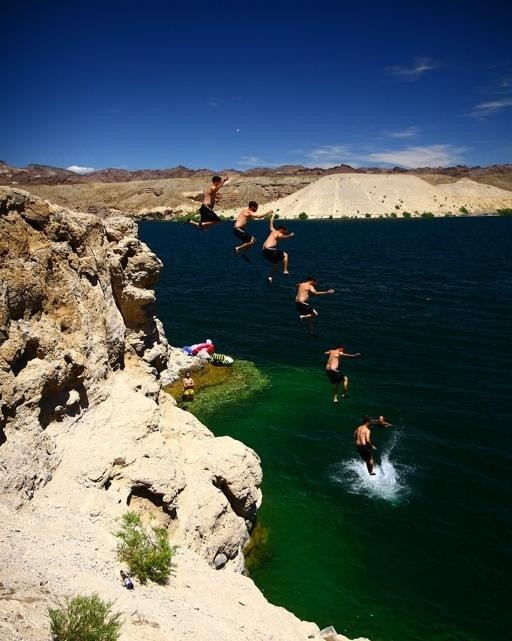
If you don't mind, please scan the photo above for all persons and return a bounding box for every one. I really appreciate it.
[185,176,229,233]
[294,279,335,322]
[352,416,378,477]
[233,201,273,257]
[262,214,295,288]
[368,415,392,430]
[323,345,362,404]
[182,371,195,401]
